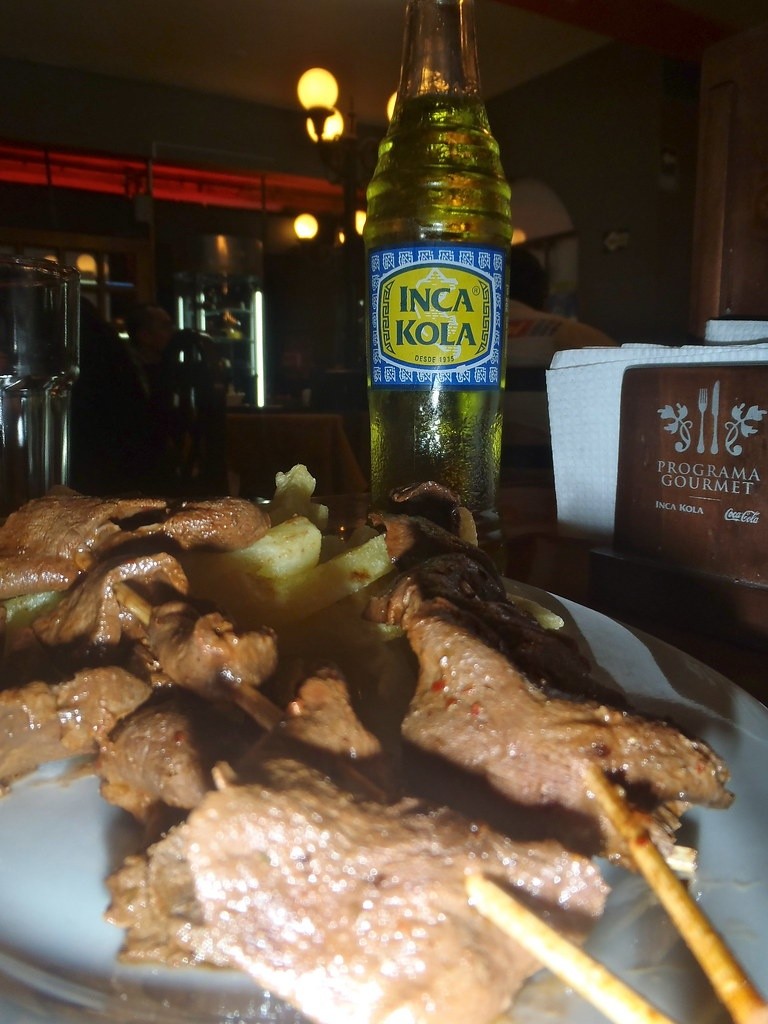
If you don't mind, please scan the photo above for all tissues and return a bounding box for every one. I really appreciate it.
[545,320,767,543]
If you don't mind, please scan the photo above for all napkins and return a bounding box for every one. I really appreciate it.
[547,342,767,540]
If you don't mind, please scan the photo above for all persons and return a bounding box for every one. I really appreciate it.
[505,245,620,470]
[0,258,248,496]
[270,340,330,405]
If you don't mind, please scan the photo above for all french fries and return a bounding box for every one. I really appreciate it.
[0,465,564,648]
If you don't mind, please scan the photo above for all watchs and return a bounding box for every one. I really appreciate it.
[233,320,241,329]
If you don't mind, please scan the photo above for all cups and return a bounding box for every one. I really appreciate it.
[0,251,83,511]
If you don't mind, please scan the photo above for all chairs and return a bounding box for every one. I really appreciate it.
[224,414,363,498]
[286,365,554,471]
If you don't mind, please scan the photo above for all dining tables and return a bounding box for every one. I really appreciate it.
[317,483,767,706]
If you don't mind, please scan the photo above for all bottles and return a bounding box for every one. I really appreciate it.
[360,0,512,579]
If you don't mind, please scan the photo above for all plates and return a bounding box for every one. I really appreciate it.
[0,590,768,1024]
